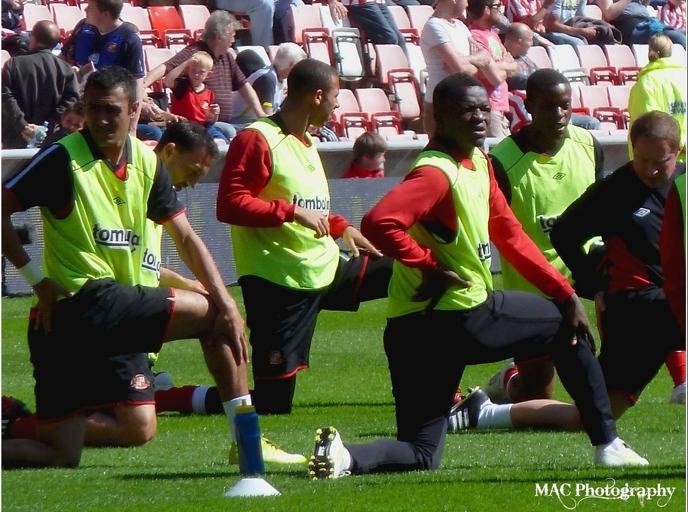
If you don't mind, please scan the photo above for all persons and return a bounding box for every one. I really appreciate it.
[656,0,686,33]
[42,99,86,150]
[153,57,464,416]
[142,12,270,141]
[484,67,609,399]
[308,73,650,482]
[325,0,406,57]
[339,132,388,180]
[504,0,583,48]
[594,0,686,50]
[467,0,519,140]
[1,67,308,470]
[445,112,686,434]
[2,118,219,448]
[165,51,228,146]
[542,0,614,45]
[57,0,145,137]
[660,175,686,338]
[628,35,688,168]
[1,21,80,149]
[231,43,306,124]
[419,0,491,139]
[502,22,600,131]
[1,0,30,56]
[136,95,188,143]
[202,1,305,53]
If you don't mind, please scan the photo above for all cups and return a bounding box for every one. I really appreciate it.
[491,3,500,9]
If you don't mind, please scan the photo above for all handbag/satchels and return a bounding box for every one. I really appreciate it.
[16,258,46,287]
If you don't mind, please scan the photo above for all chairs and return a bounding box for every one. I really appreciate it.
[448,386,489,432]
[596,437,649,466]
[229,433,307,464]
[308,426,352,481]
[671,382,686,404]
[488,361,519,402]
[153,371,173,391]
[2,395,30,439]
[1,0,688,139]
